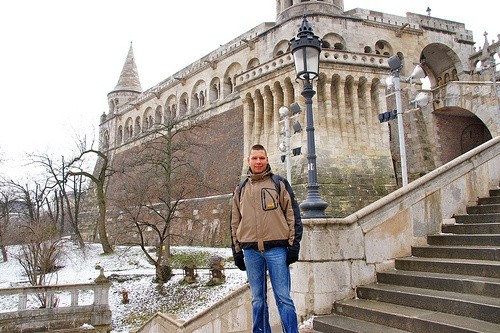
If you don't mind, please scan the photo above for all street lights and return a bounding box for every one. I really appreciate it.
[278,102,303,186]
[288,14,333,218]
[378,55,429,186]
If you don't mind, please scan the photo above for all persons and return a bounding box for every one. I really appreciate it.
[438,77,443,86]
[453,69,458,80]
[445,73,449,83]
[230,144,303,333]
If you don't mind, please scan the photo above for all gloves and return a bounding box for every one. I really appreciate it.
[286,251,299,266]
[235,254,246,271]
